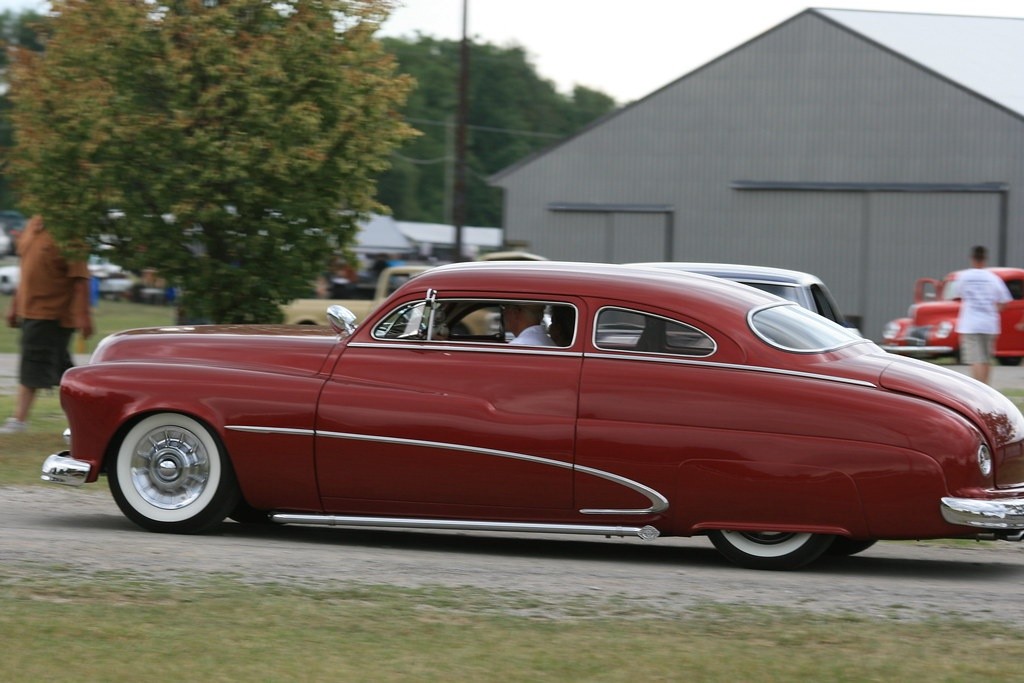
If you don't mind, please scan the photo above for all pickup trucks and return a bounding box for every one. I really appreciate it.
[279,251,550,329]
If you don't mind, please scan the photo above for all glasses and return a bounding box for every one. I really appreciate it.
[499,305,506,309]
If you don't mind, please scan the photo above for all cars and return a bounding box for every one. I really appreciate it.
[597,261,864,349]
[877,266,1024,359]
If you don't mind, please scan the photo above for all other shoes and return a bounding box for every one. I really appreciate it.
[0,417,28,434]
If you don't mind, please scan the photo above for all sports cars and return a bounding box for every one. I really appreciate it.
[37,262,1024,574]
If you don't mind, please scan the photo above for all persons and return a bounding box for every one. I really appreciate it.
[949,245,1013,383]
[497,301,557,346]
[0,213,95,436]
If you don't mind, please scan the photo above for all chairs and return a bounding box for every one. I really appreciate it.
[636,323,667,351]
